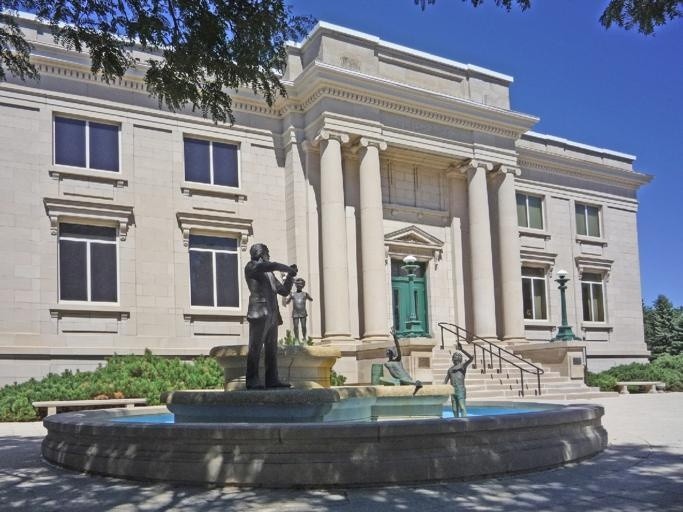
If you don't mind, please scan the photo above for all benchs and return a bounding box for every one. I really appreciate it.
[615,380,661,394]
[30,396,147,416]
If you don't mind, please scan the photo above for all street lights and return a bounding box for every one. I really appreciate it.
[551,270,582,342]
[400,255,426,337]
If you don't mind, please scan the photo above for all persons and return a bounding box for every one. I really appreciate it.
[378,325,423,388]
[443,342,474,417]
[284,278,313,341]
[244,242,298,391]
